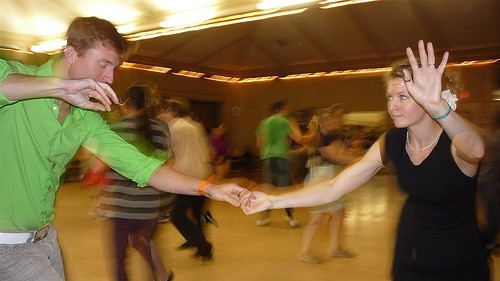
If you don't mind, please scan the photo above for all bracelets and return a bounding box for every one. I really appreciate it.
[198,180,208,195]
[430,105,451,119]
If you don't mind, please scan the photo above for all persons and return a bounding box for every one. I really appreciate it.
[81,39,500,281]
[0,17,257,281]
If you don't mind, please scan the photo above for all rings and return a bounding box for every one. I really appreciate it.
[404,78,412,82]
[428,64,436,66]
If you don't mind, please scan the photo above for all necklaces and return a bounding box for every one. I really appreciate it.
[407,128,442,151]
